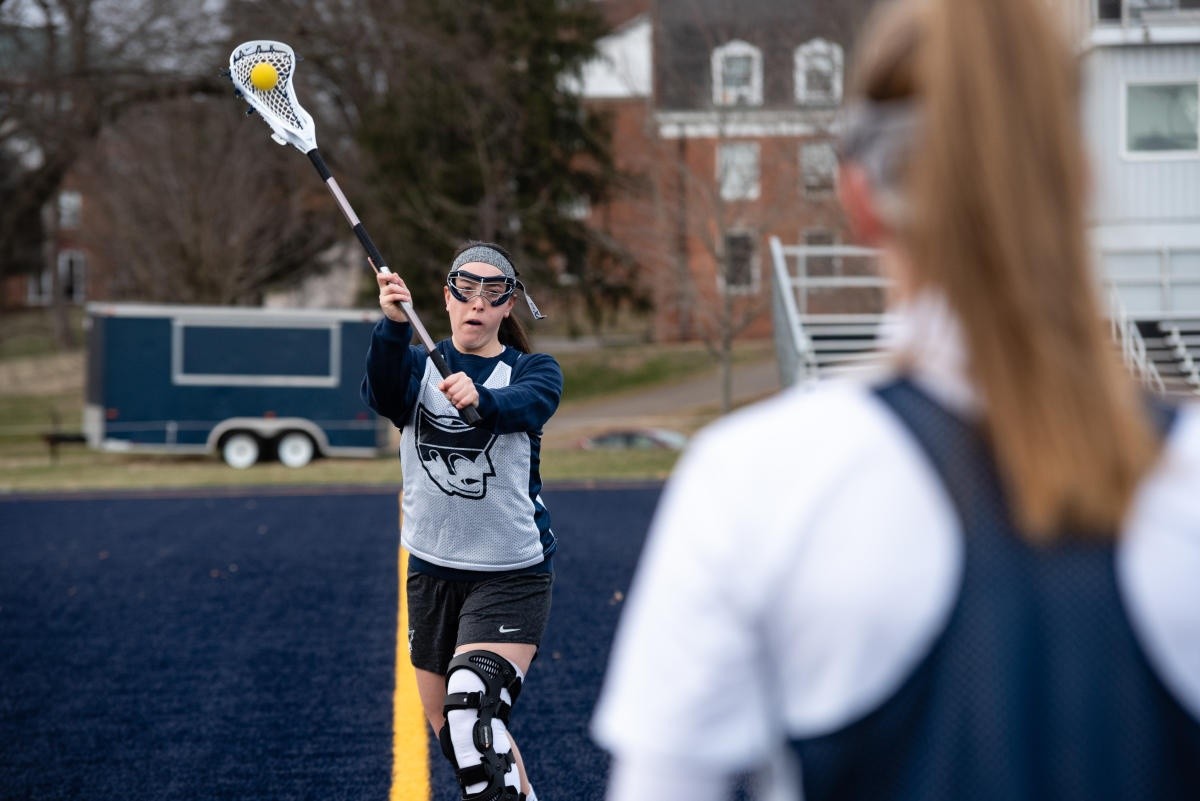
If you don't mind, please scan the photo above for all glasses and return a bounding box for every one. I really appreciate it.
[447,271,519,308]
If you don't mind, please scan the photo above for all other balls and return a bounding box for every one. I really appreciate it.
[250,62,278,89]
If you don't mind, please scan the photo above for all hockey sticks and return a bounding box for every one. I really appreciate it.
[220,40,483,426]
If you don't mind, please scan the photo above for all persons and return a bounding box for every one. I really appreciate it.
[584,0,1200,800]
[359,241,566,801]
